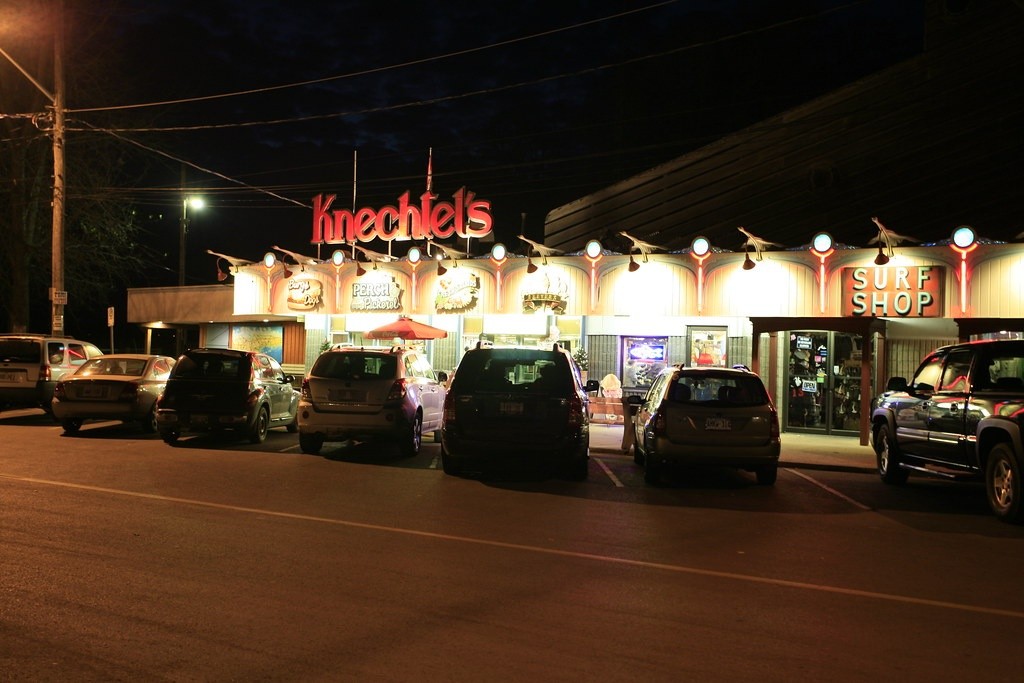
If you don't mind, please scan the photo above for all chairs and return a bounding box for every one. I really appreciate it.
[532,363,557,392]
[205,361,225,376]
[347,358,366,378]
[718,385,740,403]
[950,365,983,391]
[674,383,692,399]
[109,366,124,374]
[481,362,512,390]
[994,376,1023,392]
[379,363,396,377]
[126,366,141,376]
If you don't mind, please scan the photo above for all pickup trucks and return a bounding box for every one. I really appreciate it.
[870,338,1024,520]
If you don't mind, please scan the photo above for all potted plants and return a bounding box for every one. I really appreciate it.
[572,347,588,378]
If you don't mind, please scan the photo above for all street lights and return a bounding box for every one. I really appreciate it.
[179,195,205,287]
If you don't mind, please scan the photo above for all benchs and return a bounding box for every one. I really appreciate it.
[586,396,635,424]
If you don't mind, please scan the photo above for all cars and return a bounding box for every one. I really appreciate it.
[155,347,303,445]
[52,353,178,435]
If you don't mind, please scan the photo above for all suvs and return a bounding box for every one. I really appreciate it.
[296,346,448,459]
[441,341,600,481]
[0,333,111,414]
[626,363,780,491]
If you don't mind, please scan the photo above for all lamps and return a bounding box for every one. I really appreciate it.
[217,258,227,281]
[743,237,755,270]
[527,244,538,273]
[283,254,293,279]
[874,230,889,265]
[356,250,366,276]
[437,248,447,275]
[627,242,640,272]
